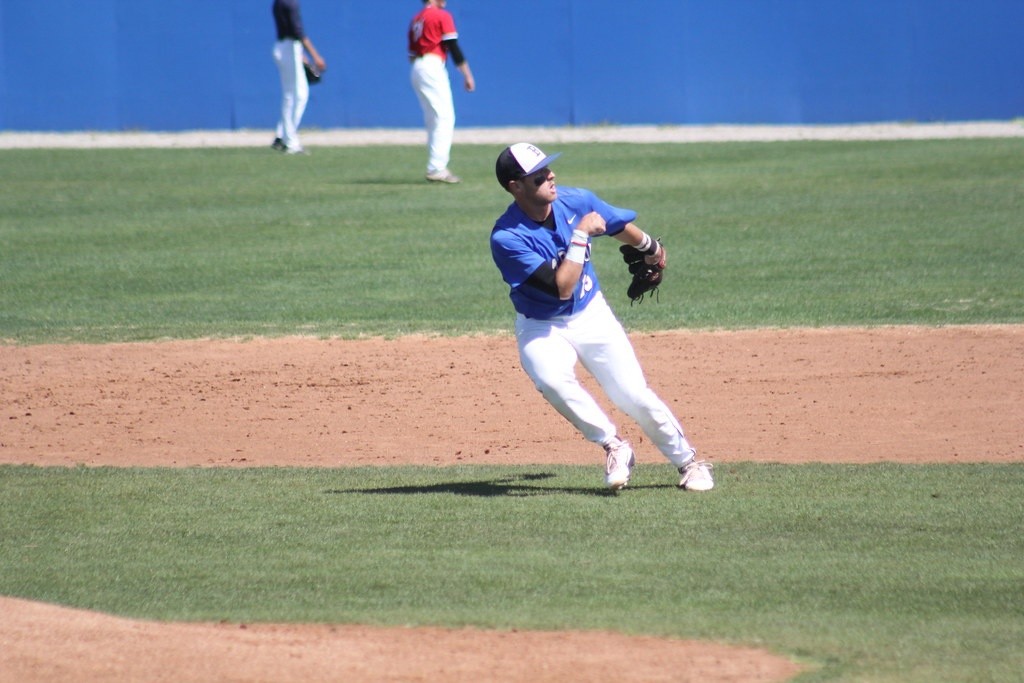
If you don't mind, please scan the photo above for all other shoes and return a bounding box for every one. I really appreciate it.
[426,169,461,183]
[270,137,288,151]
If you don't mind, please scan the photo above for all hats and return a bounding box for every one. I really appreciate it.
[494,142,561,189]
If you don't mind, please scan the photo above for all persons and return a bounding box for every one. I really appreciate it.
[270,0,326,155]
[487,142,718,495]
[405,0,476,184]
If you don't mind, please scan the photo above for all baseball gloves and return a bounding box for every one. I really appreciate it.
[619,242,665,299]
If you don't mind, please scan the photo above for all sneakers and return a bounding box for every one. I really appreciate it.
[602,436,635,491]
[678,458,714,491]
